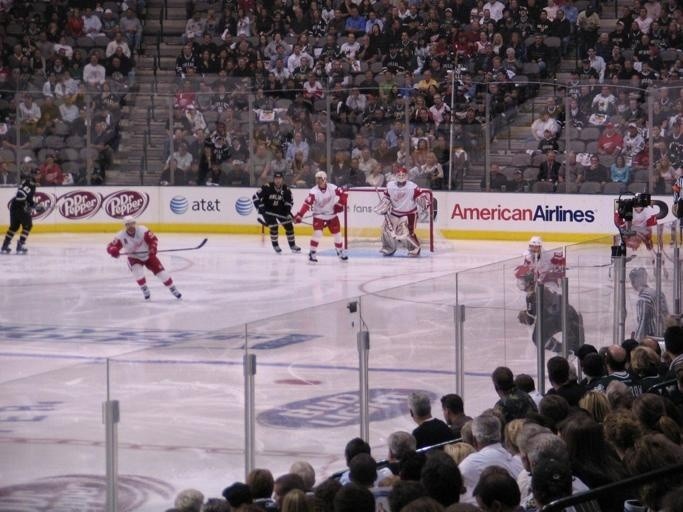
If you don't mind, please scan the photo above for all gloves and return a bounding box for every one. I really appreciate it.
[333,204,343,213]
[294,215,302,223]
[111,247,120,258]
[148,249,156,262]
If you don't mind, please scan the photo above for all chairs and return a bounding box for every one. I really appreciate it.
[157,3,683,195]
[1,0,143,186]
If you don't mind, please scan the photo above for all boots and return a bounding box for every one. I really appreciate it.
[2,241,11,250]
[309,240,319,261]
[170,285,182,298]
[335,242,348,260]
[17,241,28,251]
[141,286,150,299]
[286,233,301,250]
[271,234,282,252]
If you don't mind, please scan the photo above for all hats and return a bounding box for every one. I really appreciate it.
[24,156,32,162]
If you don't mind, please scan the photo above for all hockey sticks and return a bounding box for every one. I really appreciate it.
[375,187,410,240]
[120,239,206,255]
[564,255,634,268]
[258,213,333,228]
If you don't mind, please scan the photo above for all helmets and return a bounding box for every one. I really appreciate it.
[529,237,542,255]
[516,268,539,291]
[123,216,134,224]
[315,172,327,179]
[274,172,284,178]
[395,168,407,182]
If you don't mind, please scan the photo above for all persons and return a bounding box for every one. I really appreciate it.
[475,325,683,512]
[377,168,430,257]
[0,167,43,255]
[106,215,182,301]
[250,172,300,254]
[0,0,145,185]
[630,266,669,340]
[292,171,351,263]
[164,393,514,512]
[157,0,683,196]
[672,176,683,221]
[613,190,657,270]
[522,235,554,273]
[513,267,586,376]
